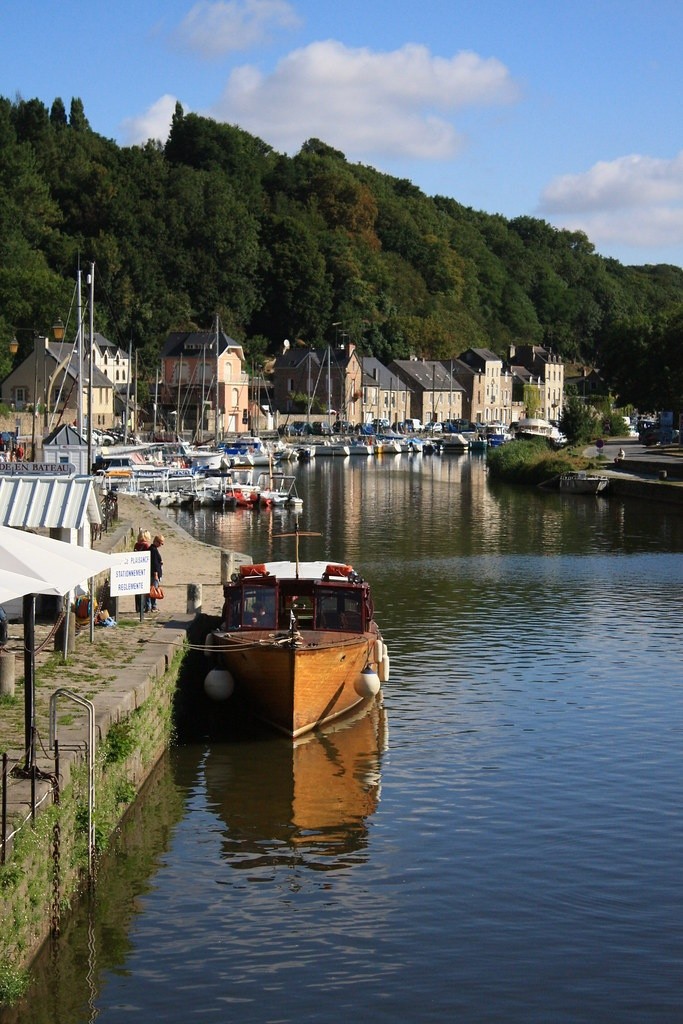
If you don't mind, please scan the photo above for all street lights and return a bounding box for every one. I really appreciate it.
[445,359,453,424]
[9,325,38,463]
[43,315,78,439]
[209,310,219,451]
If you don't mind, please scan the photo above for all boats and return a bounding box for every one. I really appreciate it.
[559,469,611,496]
[204,511,390,740]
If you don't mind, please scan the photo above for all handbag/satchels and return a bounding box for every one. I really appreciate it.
[150,579,164,599]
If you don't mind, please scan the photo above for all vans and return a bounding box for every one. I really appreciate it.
[637,419,656,434]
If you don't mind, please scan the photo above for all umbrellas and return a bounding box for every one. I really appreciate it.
[0,522,128,603]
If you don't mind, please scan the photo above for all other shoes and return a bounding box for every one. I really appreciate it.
[136,608,140,613]
[151,606,158,613]
[145,608,151,613]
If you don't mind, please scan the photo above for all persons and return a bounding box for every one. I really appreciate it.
[243,602,275,627]
[133,530,164,612]
[10,444,24,462]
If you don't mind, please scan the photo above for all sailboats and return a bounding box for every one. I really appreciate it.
[96,313,567,508]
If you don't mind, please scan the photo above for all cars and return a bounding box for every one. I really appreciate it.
[277,418,521,434]
[0,431,16,452]
[71,426,118,447]
